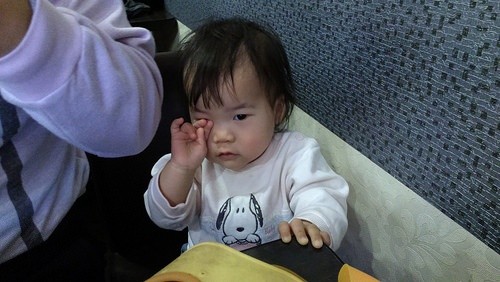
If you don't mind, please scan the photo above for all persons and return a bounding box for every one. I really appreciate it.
[144,16,351,255]
[0,1,163,282]
